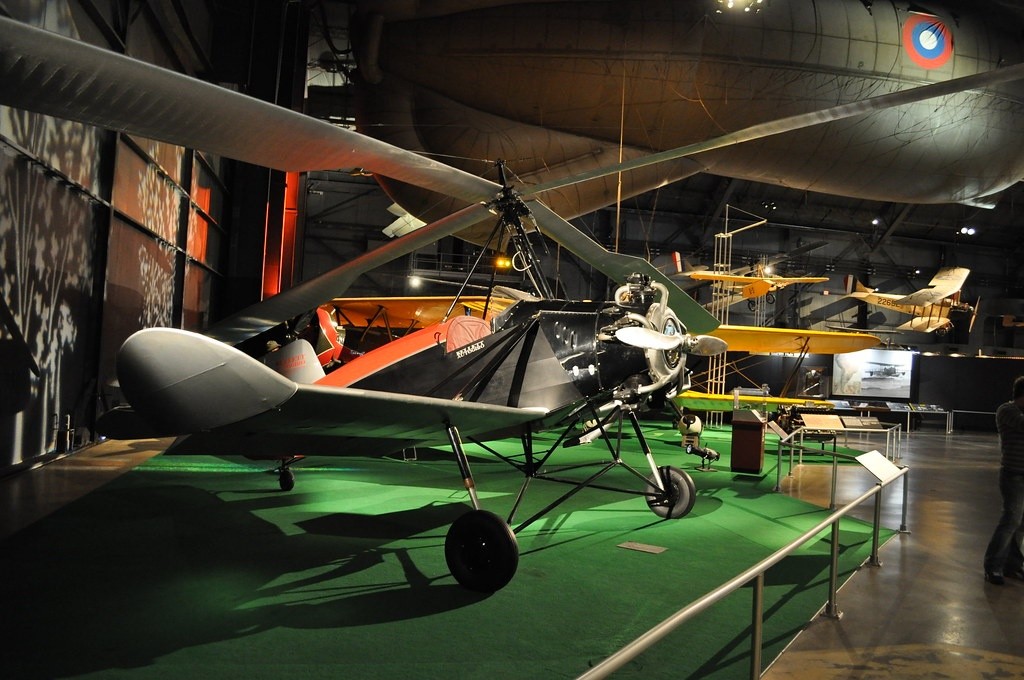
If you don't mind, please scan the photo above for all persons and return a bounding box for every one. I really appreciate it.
[984,376,1024,585]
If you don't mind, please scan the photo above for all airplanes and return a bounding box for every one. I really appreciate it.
[1,2,1023,596]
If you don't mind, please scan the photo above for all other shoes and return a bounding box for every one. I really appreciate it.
[1006,568,1024,580]
[985,571,1004,586]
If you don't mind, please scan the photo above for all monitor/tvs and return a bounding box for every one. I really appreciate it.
[828,350,920,406]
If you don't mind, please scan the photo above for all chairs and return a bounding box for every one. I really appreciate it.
[447,315,491,351]
